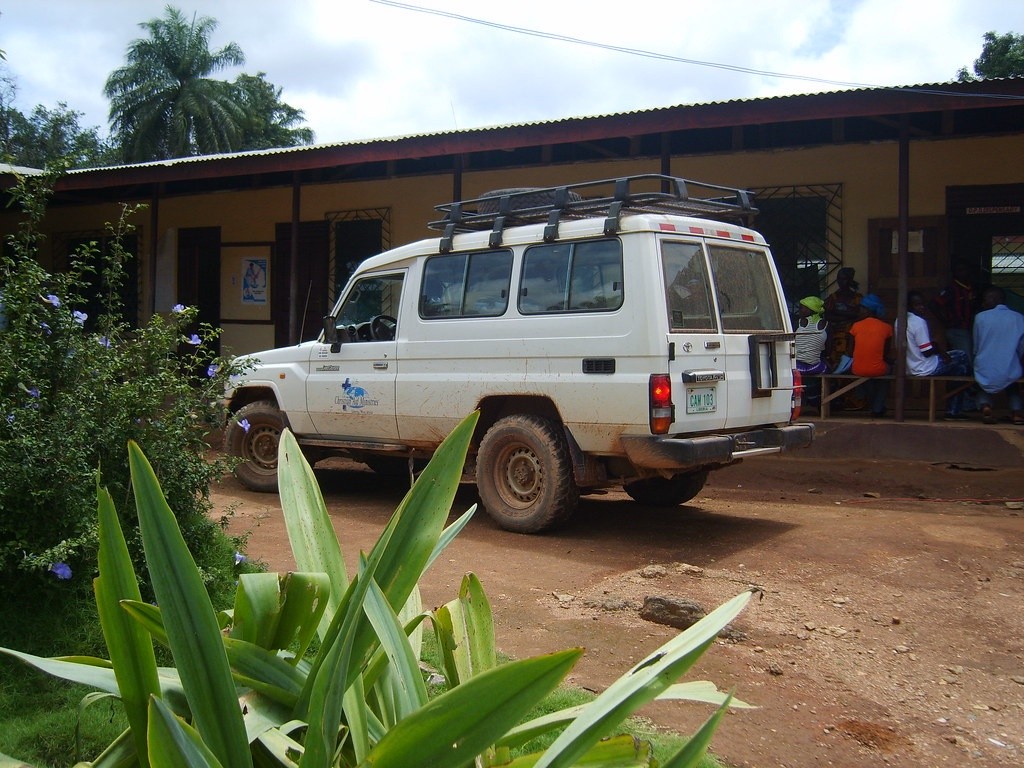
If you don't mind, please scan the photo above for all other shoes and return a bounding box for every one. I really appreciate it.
[1012,411,1024,424]
[870,404,888,417]
[984,414,996,424]
[844,398,869,410]
[943,412,972,420]
[819,408,840,416]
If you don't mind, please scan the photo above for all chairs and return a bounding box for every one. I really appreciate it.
[425,280,443,316]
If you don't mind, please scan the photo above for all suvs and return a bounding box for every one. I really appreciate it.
[218,173,816,533]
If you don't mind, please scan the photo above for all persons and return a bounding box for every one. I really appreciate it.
[847,294,894,419]
[965,287,1024,425]
[822,266,867,329]
[894,292,970,421]
[795,296,842,409]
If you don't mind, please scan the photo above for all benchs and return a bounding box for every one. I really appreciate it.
[800,373,1024,423]
